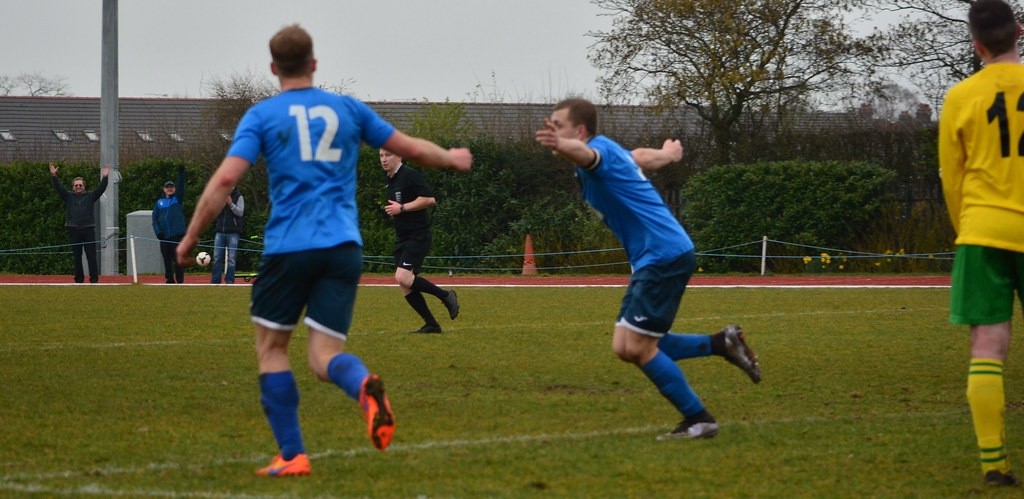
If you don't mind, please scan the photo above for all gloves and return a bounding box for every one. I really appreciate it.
[179,165,185,173]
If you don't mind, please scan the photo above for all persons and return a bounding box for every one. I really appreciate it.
[176,22,472,476]
[535,96,761,439]
[210,186,245,284]
[49,161,111,283]
[152,166,187,284]
[378,147,459,333]
[938,0,1024,486]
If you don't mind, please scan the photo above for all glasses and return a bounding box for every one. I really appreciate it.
[74,184,83,188]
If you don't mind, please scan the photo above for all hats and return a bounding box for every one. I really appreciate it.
[163,180,175,187]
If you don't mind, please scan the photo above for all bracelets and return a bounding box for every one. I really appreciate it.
[400,203,404,212]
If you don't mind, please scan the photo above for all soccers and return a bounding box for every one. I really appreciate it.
[196,252,211,266]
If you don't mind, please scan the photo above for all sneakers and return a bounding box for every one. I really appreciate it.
[358,373,395,450]
[256,452,311,477]
[442,290,460,320]
[655,411,719,441]
[984,466,1019,487]
[722,325,760,384]
[414,324,442,334]
